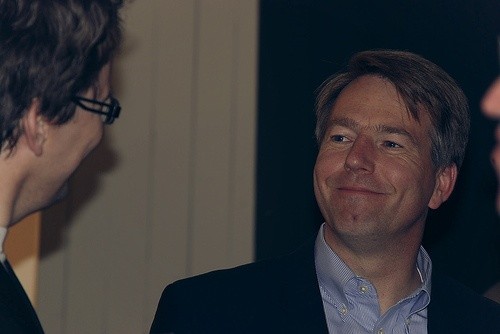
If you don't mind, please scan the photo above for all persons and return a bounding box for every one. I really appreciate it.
[0,0,126,334]
[148,49,500,334]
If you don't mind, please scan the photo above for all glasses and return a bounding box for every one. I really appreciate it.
[67,95,122,125]
